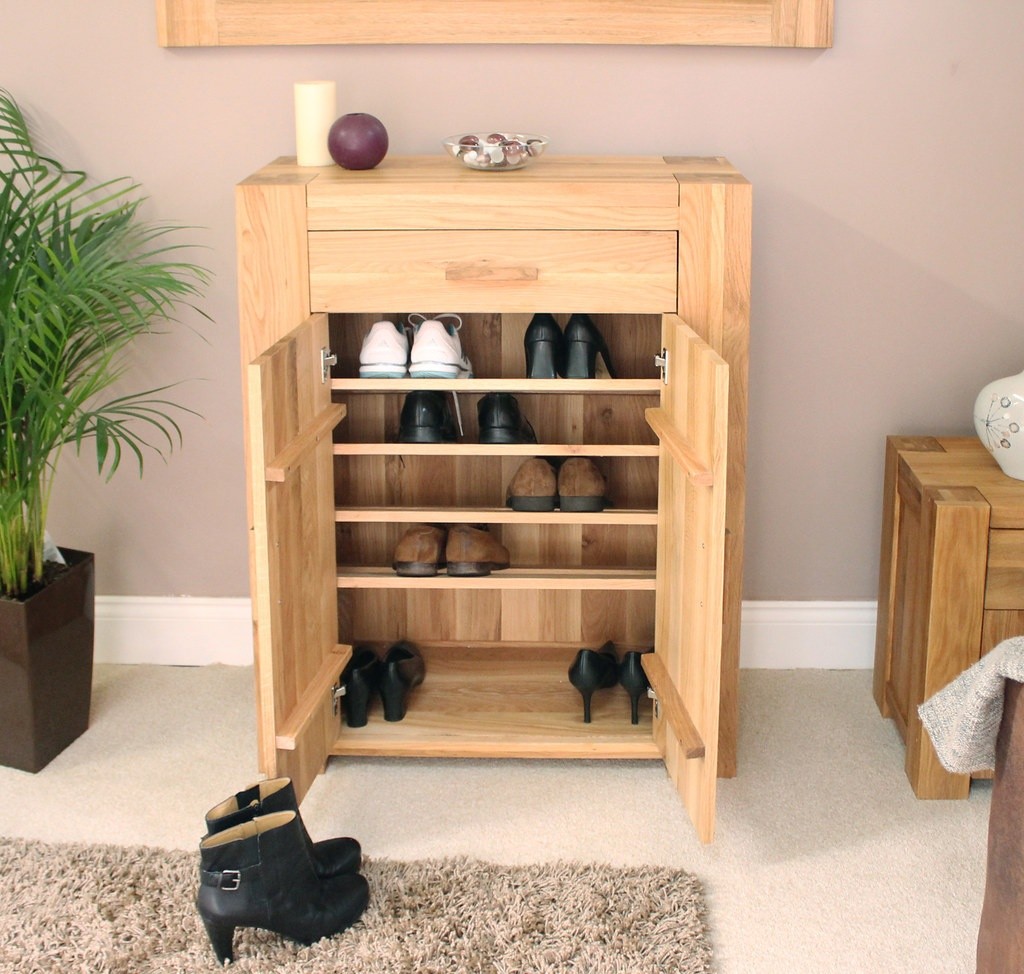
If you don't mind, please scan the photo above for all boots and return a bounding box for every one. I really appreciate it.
[196,776,370,964]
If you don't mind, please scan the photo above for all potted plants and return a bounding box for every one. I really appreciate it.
[1,77,219,775]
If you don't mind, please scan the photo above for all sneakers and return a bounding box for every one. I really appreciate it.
[359,321,411,378]
[408,314,474,378]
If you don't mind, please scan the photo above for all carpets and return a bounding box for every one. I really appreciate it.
[0,836,716,973]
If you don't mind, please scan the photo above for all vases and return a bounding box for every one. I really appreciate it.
[973,368,1023,484]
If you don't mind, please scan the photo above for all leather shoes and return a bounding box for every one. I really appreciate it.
[393,524,446,577]
[558,457,613,513]
[400,392,458,443]
[476,393,539,444]
[506,458,557,511]
[447,524,510,576]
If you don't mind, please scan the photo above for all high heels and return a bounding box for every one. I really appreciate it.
[339,647,379,727]
[524,313,619,379]
[376,640,426,721]
[567,640,619,722]
[619,647,655,725]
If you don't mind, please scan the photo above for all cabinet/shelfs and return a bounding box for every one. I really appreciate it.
[871,434,1023,799]
[234,155,756,849]
